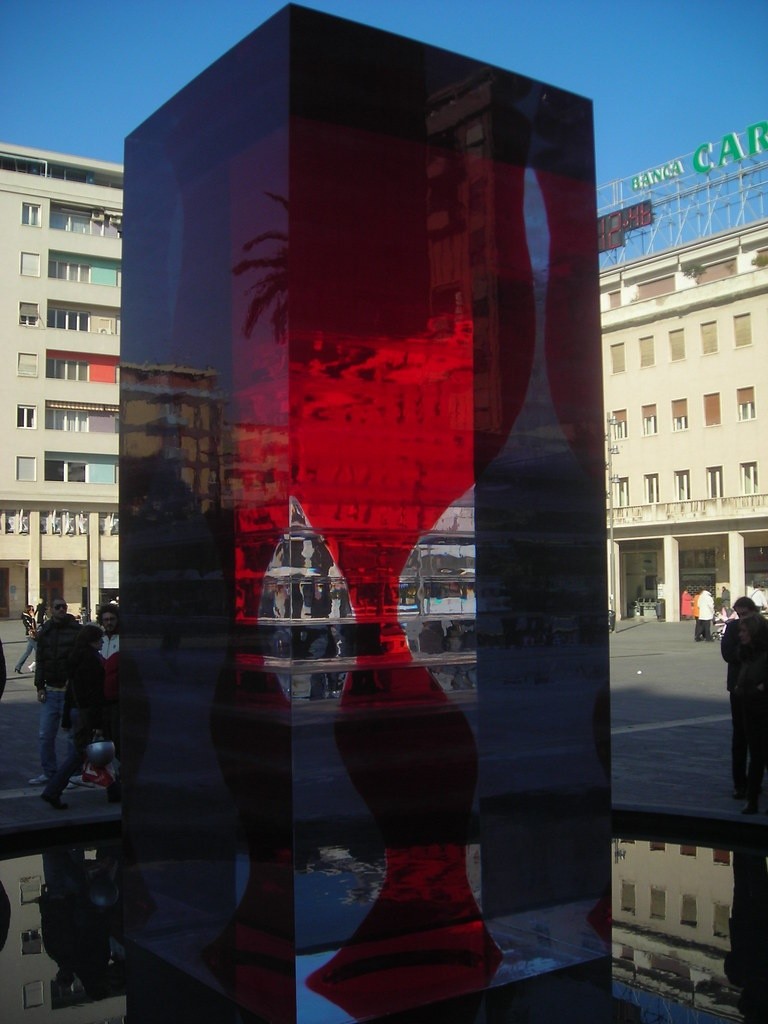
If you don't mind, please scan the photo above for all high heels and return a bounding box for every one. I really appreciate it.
[14,668,23,674]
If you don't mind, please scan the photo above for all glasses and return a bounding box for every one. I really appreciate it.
[52,605,67,610]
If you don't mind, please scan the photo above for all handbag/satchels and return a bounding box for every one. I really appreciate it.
[28,629,38,640]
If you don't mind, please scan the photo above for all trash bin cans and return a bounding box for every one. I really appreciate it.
[608,610,616,632]
[655,598,665,620]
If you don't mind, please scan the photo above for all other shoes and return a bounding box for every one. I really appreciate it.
[28,774,51,785]
[106,784,123,804]
[41,785,68,810]
[70,775,95,788]
[66,782,77,789]
[741,801,759,815]
[733,790,744,799]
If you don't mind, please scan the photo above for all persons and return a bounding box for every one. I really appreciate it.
[261,501,354,701]
[0,596,121,810]
[681,583,767,642]
[720,596,767,815]
